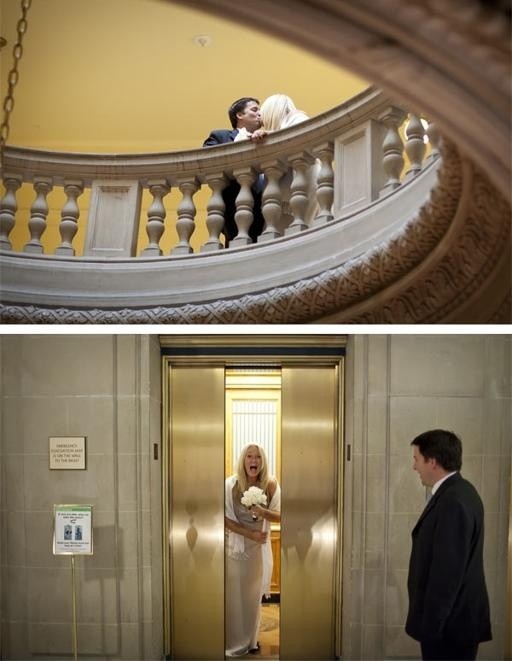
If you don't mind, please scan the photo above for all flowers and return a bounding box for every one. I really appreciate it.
[241,484,272,518]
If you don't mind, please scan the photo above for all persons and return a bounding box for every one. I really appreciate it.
[403,430,488,660]
[201,93,264,250]
[224,442,282,660]
[251,92,324,227]
